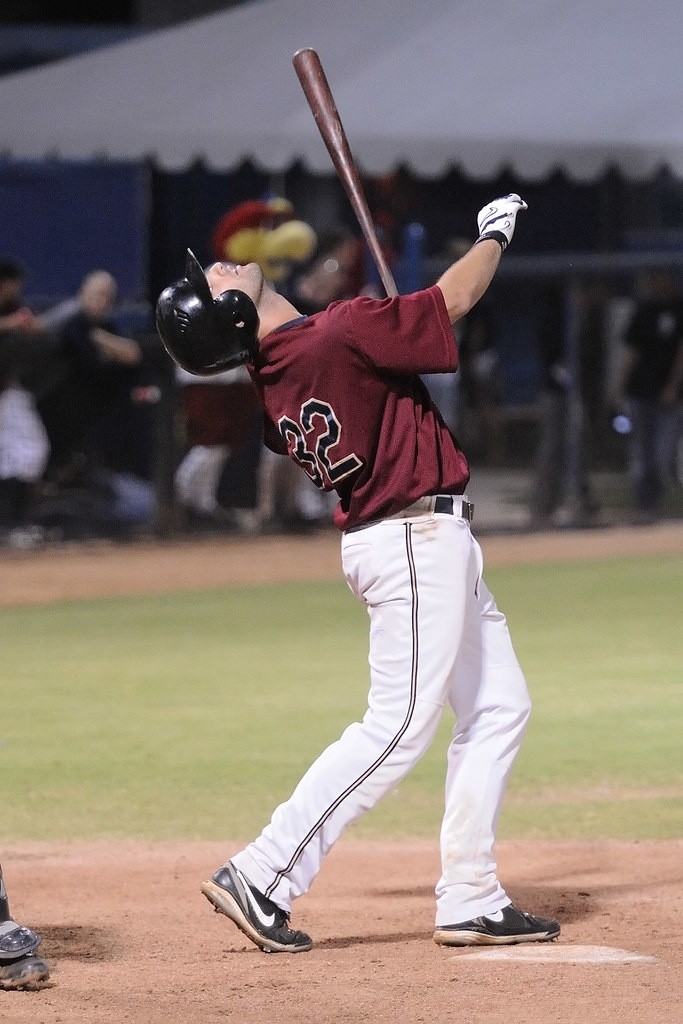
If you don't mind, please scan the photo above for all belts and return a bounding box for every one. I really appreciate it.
[433,497,476,524]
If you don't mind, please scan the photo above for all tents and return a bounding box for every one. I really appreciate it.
[0,0,683,518]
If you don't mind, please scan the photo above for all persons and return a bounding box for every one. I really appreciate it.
[0,212,683,544]
[0,866,49,990]
[175,191,561,951]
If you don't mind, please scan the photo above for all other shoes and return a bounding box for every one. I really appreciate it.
[0,869,53,989]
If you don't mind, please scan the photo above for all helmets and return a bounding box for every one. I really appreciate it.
[156,247,260,376]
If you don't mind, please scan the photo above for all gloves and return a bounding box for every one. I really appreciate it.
[473,192,528,250]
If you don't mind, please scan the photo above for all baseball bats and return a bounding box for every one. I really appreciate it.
[287,40,406,304]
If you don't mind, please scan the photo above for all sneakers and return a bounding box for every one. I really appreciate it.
[199,859,312,952]
[434,903,560,947]
